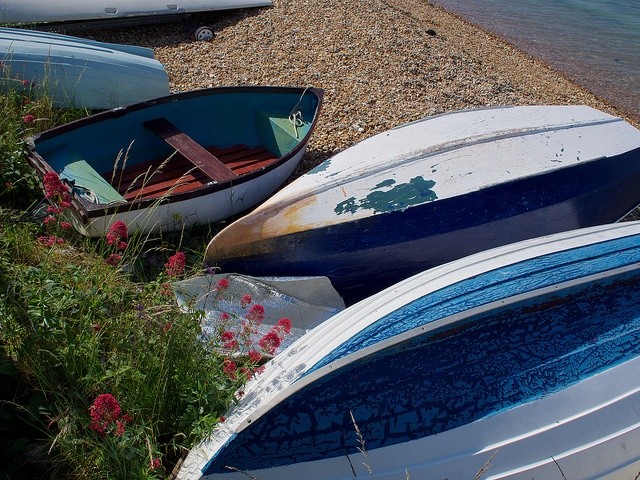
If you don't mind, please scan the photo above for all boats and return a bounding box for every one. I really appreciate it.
[0,26,170,110]
[0,0,273,42]
[203,104,639,295]
[23,85,323,238]
[165,221,640,479]
